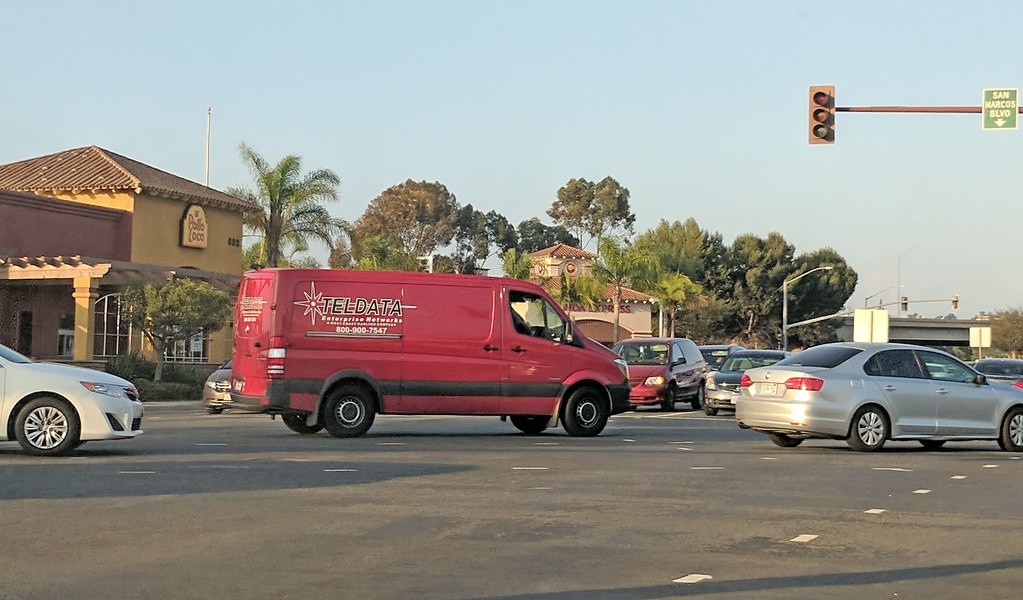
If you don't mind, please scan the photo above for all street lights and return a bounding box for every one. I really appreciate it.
[864,283,904,308]
[783,266,832,350]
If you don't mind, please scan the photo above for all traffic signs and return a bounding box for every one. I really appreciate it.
[982,88,1019,129]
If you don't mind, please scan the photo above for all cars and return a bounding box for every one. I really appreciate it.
[608,337,708,412]
[697,345,754,374]
[0,345,144,455]
[704,350,791,416]
[925,361,975,383]
[201,356,232,414]
[968,357,1023,388]
[736,340,1023,454]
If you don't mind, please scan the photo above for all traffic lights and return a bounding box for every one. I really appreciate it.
[807,85,836,145]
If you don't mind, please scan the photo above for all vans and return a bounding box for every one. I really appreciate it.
[232,264,639,441]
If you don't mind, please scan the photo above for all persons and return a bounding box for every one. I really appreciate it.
[655,350,667,362]
